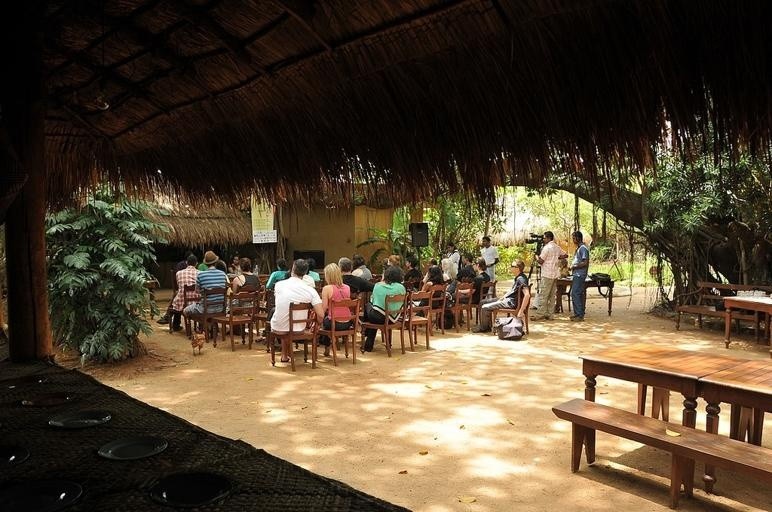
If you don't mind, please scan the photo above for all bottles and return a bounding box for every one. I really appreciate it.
[254,265,259,276]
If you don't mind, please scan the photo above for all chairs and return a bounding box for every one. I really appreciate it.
[471,280,497,325]
[492,283,533,335]
[318,299,361,366]
[167,271,424,342]
[361,292,409,357]
[405,290,434,352]
[271,302,318,371]
[556,275,574,312]
[446,281,475,333]
[424,283,448,336]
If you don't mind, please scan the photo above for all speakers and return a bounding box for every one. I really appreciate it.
[409,223,428,247]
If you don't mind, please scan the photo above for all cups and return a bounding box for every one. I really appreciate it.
[737,290,766,299]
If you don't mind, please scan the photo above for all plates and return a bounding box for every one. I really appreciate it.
[145,470,233,510]
[18,389,84,411]
[48,409,111,429]
[5,376,49,390]
[0,444,32,474]
[14,481,83,512]
[96,434,168,462]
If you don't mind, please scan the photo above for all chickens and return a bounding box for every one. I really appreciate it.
[191,331,206,356]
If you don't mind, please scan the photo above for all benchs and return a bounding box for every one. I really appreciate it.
[674,281,772,344]
[552,399,772,512]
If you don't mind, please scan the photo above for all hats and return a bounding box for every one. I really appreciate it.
[203,251,219,265]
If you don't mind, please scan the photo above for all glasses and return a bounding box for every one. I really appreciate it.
[511,264,519,268]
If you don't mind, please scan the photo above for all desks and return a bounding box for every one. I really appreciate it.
[699,358,772,493]
[556,277,614,316]
[130,281,156,320]
[723,296,772,358]
[578,334,751,493]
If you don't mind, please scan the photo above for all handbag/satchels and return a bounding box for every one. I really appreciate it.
[592,273,611,296]
[492,316,523,339]
[353,319,362,332]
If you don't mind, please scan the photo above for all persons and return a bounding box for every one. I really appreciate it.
[526,255,540,310]
[440,242,460,283]
[553,257,568,313]
[568,231,589,322]
[157,250,491,361]
[529,230,567,321]
[470,259,531,333]
[479,237,499,298]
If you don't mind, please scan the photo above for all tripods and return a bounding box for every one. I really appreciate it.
[527,242,541,294]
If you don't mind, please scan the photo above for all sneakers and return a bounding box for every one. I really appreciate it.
[473,328,489,333]
[173,326,182,331]
[158,316,169,324]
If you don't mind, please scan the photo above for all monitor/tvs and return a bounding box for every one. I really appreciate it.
[294,250,325,269]
